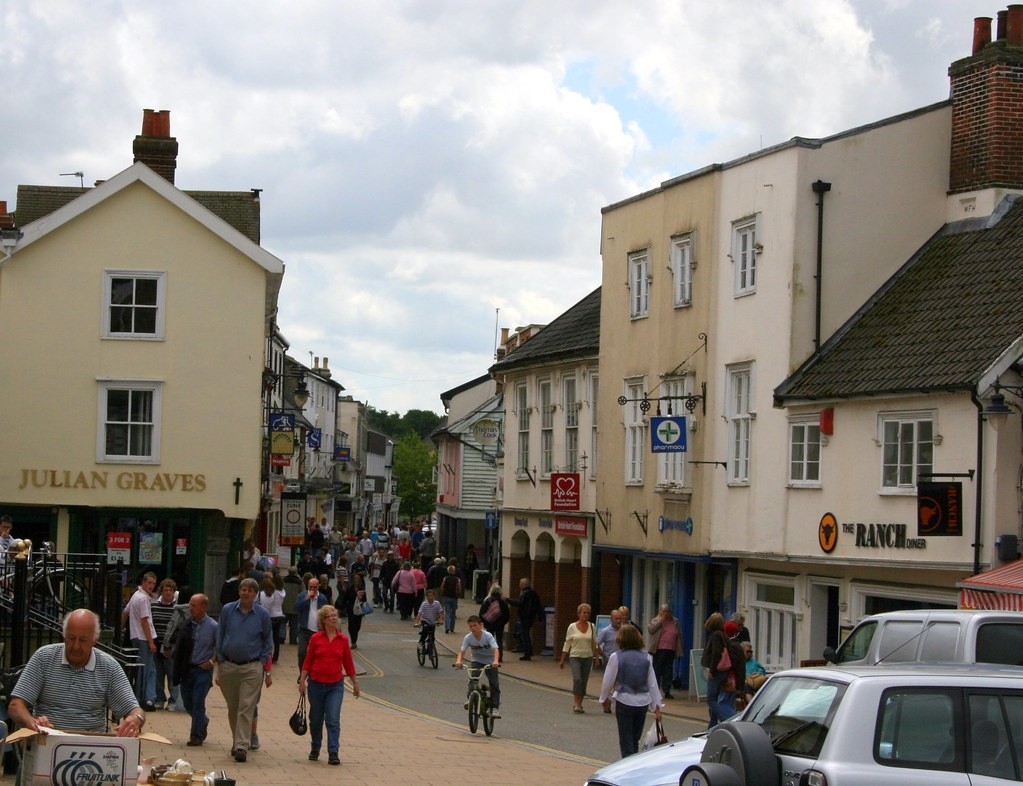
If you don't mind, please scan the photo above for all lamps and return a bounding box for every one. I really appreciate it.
[981,373,1023,433]
[262,371,312,408]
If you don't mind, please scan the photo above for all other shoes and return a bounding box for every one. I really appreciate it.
[144,703,156,712]
[328,752,340,765]
[165,703,179,711]
[154,701,164,708]
[231,746,235,756]
[234,749,246,762]
[251,734,259,748]
[203,718,209,741]
[309,745,321,760]
[187,737,203,746]
[464,701,469,710]
[573,705,584,713]
[520,655,531,661]
[351,644,357,649]
[511,648,523,653]
[491,708,500,716]
[603,699,611,713]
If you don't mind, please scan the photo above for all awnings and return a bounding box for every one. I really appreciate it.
[961,559,1023,611]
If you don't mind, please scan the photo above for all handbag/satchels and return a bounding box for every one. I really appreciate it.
[353,596,373,616]
[642,718,668,751]
[289,692,307,735]
[482,598,501,623]
[393,571,401,592]
[745,673,768,689]
[716,631,732,670]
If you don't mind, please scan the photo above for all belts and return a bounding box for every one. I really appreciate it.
[224,656,260,666]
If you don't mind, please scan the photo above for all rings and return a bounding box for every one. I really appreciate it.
[129,729,134,733]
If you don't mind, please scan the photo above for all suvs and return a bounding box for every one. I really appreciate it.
[680,664,1023,786]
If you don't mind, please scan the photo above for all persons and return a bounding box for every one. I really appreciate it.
[242,517,479,684]
[479,579,543,667]
[455,615,500,718]
[0,521,16,600]
[598,624,665,759]
[8,608,145,737]
[701,612,766,729]
[647,604,684,700]
[560,604,609,713]
[299,605,359,765]
[35,542,67,597]
[120,571,272,762]
[598,607,643,669]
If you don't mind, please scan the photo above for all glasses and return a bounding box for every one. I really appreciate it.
[745,650,753,654]
[309,586,317,589]
[324,614,338,618]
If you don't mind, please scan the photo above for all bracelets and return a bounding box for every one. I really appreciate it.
[209,659,214,667]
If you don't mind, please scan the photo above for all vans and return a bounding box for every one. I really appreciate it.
[823,609,1023,669]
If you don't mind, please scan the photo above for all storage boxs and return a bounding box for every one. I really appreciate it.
[5,721,174,786]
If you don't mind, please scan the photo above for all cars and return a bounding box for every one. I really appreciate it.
[422,522,436,536]
[582,704,751,786]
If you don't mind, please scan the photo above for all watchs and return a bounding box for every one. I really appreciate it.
[128,713,144,726]
[266,671,271,676]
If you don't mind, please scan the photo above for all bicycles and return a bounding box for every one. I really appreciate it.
[451,663,501,736]
[0,541,90,623]
[413,619,444,669]
